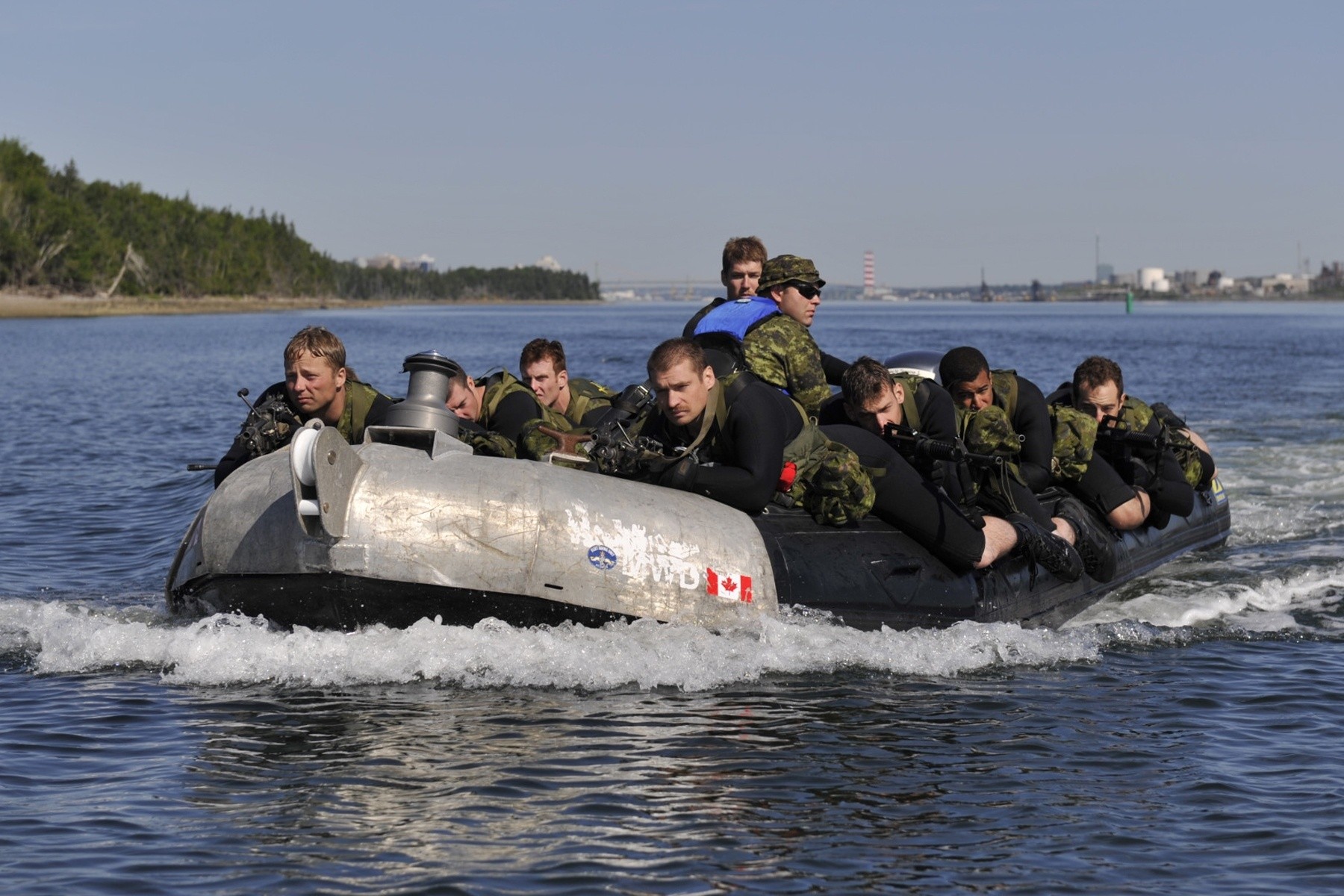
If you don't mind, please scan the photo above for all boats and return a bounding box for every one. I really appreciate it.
[165,349,1229,651]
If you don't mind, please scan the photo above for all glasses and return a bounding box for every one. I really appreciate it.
[790,282,822,300]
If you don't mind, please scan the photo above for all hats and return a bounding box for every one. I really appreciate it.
[755,255,826,297]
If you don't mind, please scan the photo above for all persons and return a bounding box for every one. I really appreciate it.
[213,236,1219,585]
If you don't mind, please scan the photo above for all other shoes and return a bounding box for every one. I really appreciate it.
[1150,402,1190,430]
[1129,457,1159,492]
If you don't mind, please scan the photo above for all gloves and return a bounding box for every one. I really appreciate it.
[907,454,950,488]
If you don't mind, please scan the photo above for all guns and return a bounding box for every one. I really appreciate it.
[1097,414,1198,469]
[230,388,298,459]
[1017,433,1026,442]
[589,426,689,476]
[187,463,218,471]
[879,421,1004,471]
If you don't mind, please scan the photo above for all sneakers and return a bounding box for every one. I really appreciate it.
[1055,498,1117,584]
[1004,513,1085,592]
